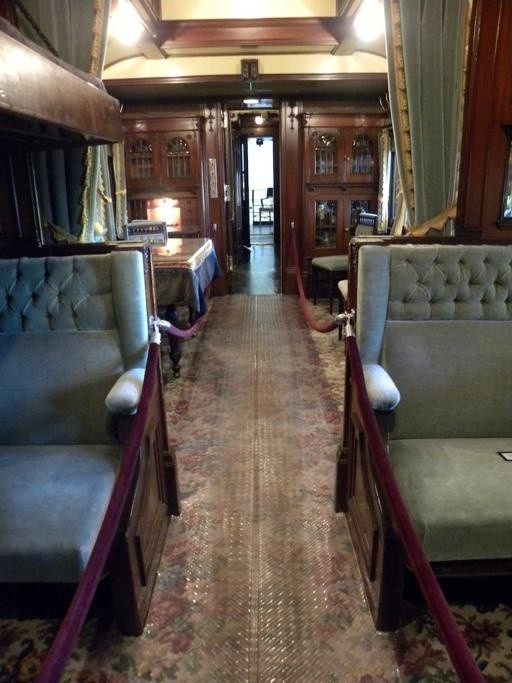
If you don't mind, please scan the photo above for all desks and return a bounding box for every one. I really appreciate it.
[152,237,214,378]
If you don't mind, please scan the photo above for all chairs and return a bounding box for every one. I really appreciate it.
[311,212,377,316]
[259,187,273,227]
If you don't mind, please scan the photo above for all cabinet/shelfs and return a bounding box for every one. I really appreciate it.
[299,113,384,299]
[121,115,211,238]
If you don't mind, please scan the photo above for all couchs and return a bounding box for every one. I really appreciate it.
[334,233,512,638]
[0,238,183,639]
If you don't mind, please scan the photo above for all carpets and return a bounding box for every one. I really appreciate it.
[250,232,275,245]
